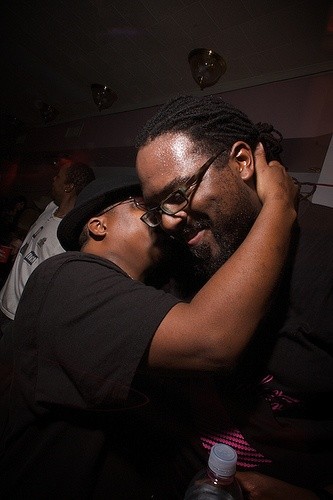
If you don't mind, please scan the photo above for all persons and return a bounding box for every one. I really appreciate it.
[0,142,300,500]
[0,160,96,337]
[136,95,333,500]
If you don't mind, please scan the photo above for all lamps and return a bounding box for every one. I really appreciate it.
[91,82,118,113]
[188,48,227,87]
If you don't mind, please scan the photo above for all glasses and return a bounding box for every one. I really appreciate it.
[138,145,232,229]
[92,192,149,218]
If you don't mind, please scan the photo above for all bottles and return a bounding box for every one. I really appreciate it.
[185,444,243,500]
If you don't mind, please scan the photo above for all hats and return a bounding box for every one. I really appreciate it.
[56,174,142,253]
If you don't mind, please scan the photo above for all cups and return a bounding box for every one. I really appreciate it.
[0,245,11,263]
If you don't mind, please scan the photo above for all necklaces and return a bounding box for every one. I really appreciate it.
[128,273,135,281]
